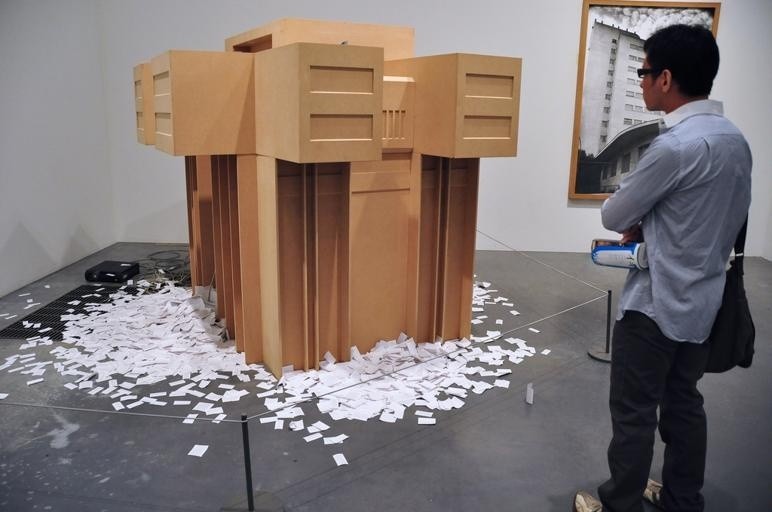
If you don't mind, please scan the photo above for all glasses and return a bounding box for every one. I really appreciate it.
[637,68,653,79]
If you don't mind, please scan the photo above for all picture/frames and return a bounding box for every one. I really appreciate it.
[568,0,722,204]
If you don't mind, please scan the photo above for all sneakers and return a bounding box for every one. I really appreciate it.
[642,477,664,504]
[575,490,602,511]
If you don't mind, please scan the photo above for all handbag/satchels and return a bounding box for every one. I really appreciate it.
[703,254,756,373]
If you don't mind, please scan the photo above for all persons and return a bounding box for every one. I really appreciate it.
[573,22,755,511]
